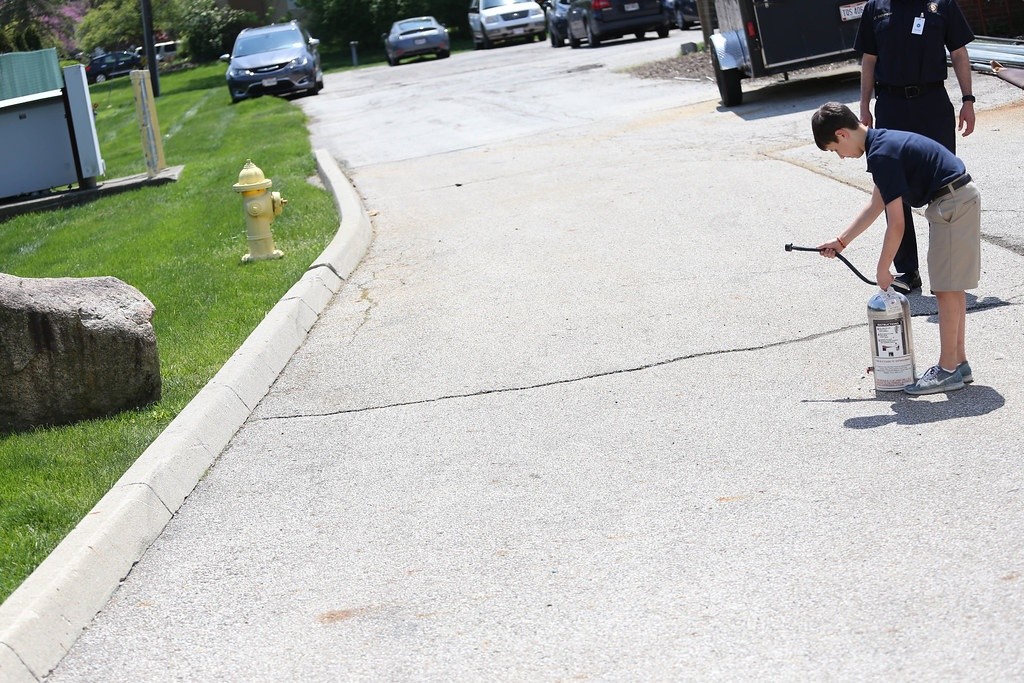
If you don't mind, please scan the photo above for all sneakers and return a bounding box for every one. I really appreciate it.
[904,364,965,395]
[958,361,974,384]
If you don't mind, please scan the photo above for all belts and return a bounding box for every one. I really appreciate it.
[934,173,971,198]
[872,81,945,99]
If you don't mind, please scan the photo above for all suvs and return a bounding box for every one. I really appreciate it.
[219,19,324,104]
[84,50,146,85]
[467,0,548,51]
[709,0,869,108]
[135,40,178,63]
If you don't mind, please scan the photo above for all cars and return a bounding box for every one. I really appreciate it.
[543,0,720,49]
[379,15,451,68]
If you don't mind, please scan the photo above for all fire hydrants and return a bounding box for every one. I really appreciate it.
[231,158,288,262]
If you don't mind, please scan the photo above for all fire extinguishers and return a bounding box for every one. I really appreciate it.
[785,243,917,392]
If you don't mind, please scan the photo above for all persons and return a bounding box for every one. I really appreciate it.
[854,0,975,297]
[811,100,981,396]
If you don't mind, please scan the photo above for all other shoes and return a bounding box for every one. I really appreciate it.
[889,269,922,296]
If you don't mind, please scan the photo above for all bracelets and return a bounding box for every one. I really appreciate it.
[962,95,975,103]
[836,237,847,249]
